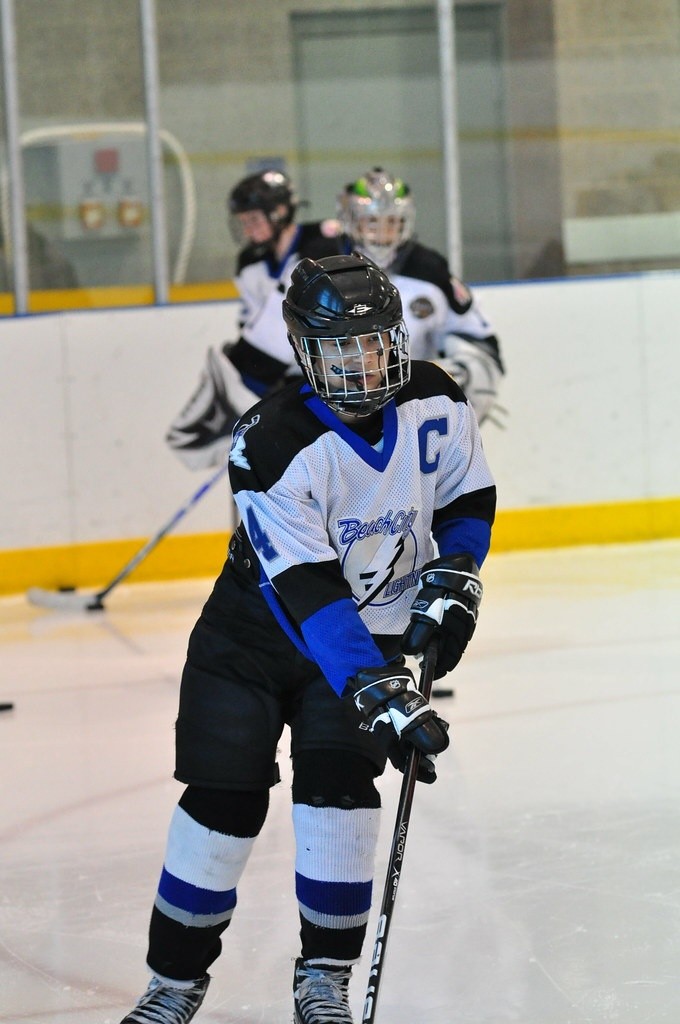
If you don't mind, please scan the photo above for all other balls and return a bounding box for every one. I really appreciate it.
[1,700,16,712]
[56,583,75,593]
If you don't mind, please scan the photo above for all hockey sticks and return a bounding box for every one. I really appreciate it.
[28,450,229,611]
[362,643,437,1024]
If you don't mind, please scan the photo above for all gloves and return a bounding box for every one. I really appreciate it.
[401,553,482,680]
[350,669,449,784]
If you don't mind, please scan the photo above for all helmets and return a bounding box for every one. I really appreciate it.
[282,252,411,416]
[339,166,413,267]
[229,168,292,213]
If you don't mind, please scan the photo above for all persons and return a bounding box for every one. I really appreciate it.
[166,167,508,475]
[120,250,496,1024]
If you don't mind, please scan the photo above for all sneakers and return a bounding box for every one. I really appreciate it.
[118,975,210,1024]
[291,956,359,1024]
